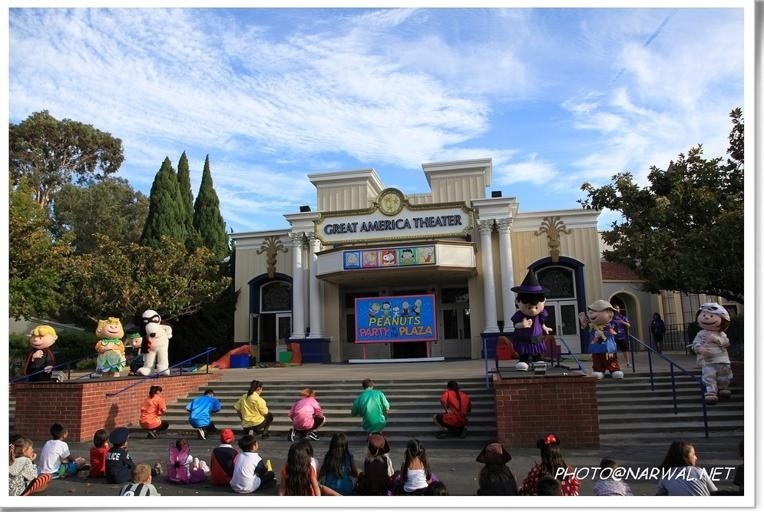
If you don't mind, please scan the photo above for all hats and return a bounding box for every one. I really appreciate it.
[475,443,512,466]
[368,429,391,456]
[109,427,129,445]
[219,427,235,441]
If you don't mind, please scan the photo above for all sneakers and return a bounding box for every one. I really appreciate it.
[81,464,91,471]
[287,428,321,444]
[196,427,207,440]
[626,360,634,371]
[433,425,471,440]
[154,463,164,475]
[248,427,273,440]
[144,430,160,440]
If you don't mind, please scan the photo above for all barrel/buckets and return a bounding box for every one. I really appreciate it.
[279,351,293,363]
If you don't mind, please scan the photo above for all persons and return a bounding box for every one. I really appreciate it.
[186,389,222,441]
[351,378,390,437]
[433,380,472,438]
[651,312,666,355]
[287,387,326,442]
[233,379,273,439]
[138,385,170,439]
[608,305,632,368]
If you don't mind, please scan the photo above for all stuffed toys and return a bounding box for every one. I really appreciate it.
[95,309,173,378]
[690,302,734,403]
[19,325,59,381]
[579,299,624,380]
[509,268,554,372]
